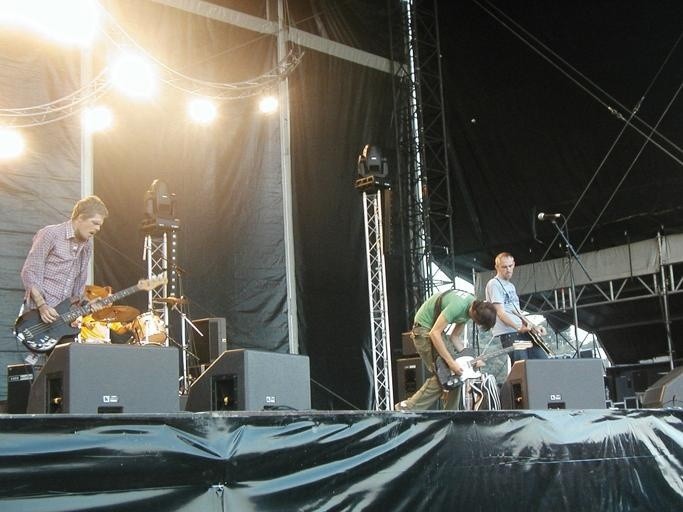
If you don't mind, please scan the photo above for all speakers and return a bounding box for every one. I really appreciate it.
[27,341,180,415]
[185,348,312,412]
[396,357,432,402]
[644,366,683,408]
[501,358,607,409]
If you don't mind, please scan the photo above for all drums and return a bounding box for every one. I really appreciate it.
[131,311,167,343]
[141,342,164,347]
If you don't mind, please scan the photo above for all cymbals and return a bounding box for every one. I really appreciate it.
[153,297,187,304]
[92,306,137,324]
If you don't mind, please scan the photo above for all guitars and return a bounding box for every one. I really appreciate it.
[15,275,167,355]
[512,310,551,356]
[435,340,534,390]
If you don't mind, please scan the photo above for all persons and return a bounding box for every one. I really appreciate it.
[394,289,497,411]
[20,196,109,385]
[485,253,549,370]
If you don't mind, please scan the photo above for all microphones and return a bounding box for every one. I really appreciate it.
[537,212,562,220]
